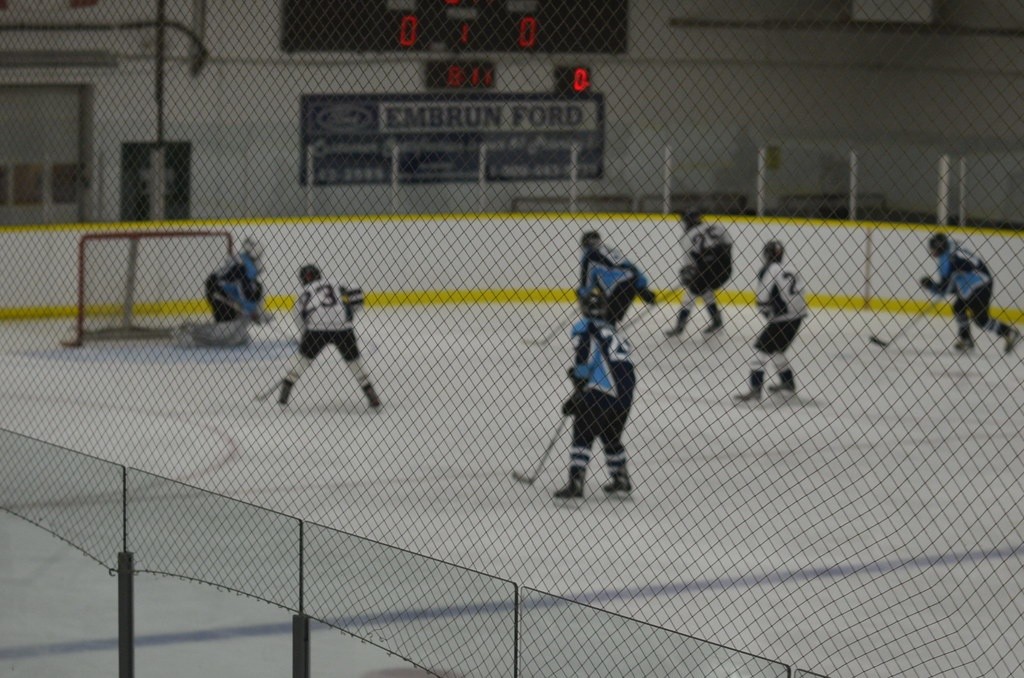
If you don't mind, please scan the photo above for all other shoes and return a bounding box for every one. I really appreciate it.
[996,322,1019,352]
[954,325,974,350]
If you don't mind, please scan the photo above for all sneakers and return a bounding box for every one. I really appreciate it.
[279,379,292,404]
[704,303,722,332]
[603,446,632,498]
[665,309,690,336]
[361,384,380,407]
[735,370,764,401]
[553,445,590,508]
[768,370,795,392]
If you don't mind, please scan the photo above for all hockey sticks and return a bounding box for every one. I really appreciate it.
[870,294,945,349]
[524,305,582,346]
[511,412,568,485]
[252,373,287,402]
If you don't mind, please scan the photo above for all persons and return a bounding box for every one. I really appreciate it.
[665,200,734,336]
[919,232,1019,353]
[554,230,656,499]
[734,240,806,401]
[180,239,272,348]
[277,265,382,407]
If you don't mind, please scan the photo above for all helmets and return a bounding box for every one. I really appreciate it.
[680,207,701,231]
[929,233,949,258]
[763,241,783,262]
[581,232,601,250]
[298,264,321,285]
[581,293,608,320]
